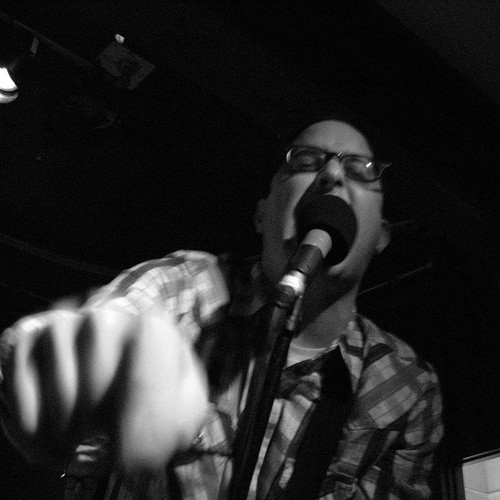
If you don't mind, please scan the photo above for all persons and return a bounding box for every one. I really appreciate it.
[1,115,445,500]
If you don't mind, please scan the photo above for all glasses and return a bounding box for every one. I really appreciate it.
[282,144,392,182]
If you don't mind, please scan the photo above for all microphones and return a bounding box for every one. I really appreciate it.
[273,195,357,308]
